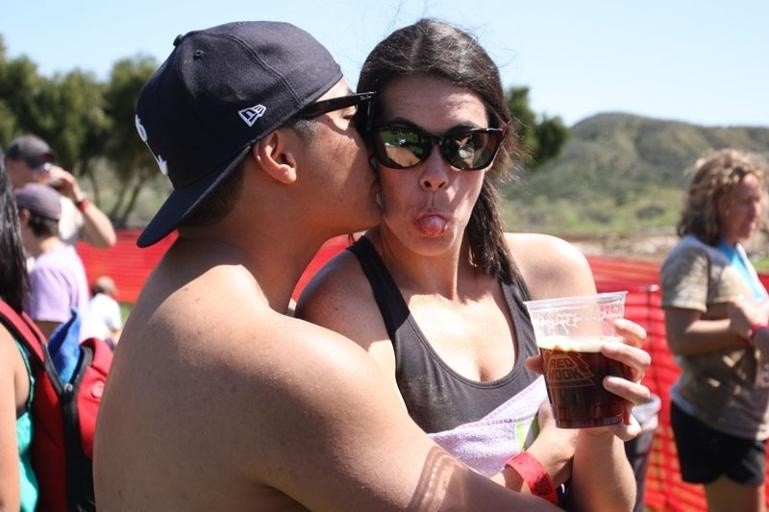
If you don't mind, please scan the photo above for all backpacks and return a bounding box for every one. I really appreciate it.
[0,300,113,512]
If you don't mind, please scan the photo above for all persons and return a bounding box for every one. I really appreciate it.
[659,150,769,512]
[295,20,651,511]
[1,135,122,511]
[94,21,565,511]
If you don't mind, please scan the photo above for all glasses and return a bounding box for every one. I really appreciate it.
[358,116,506,172]
[289,89,376,145]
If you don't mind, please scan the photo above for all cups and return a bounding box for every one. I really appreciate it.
[520,293,627,432]
[31,160,67,191]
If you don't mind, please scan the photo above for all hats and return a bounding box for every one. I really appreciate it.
[0,134,51,170]
[133,21,343,247]
[13,182,61,220]
